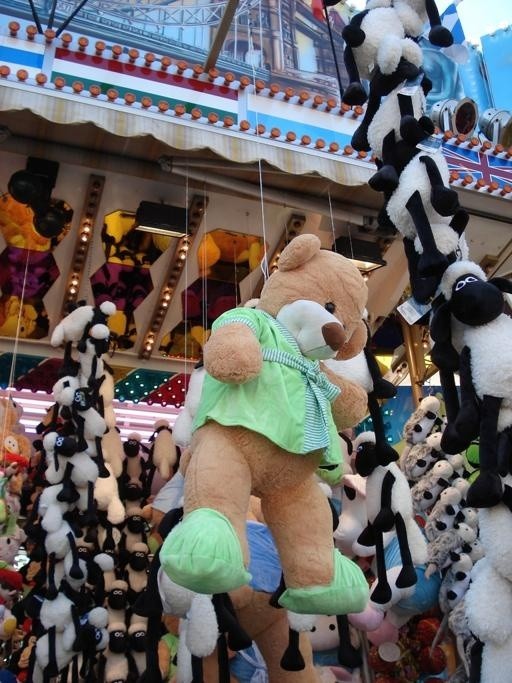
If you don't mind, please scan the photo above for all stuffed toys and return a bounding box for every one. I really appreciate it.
[0,149,512,682]
[0,149,511,682]
[339,0,452,194]
